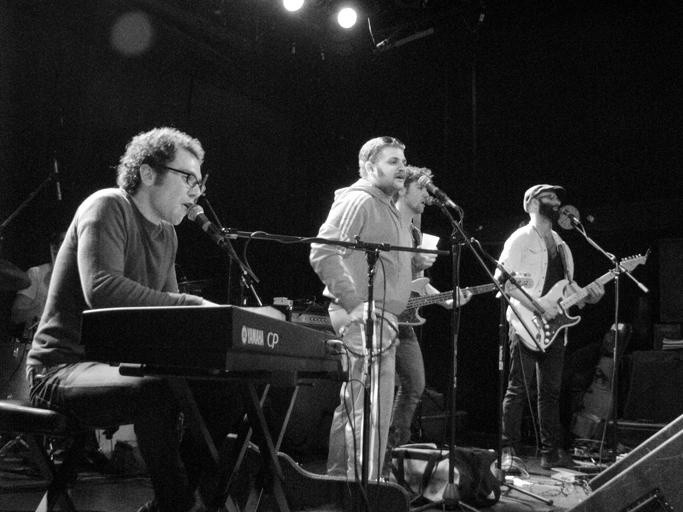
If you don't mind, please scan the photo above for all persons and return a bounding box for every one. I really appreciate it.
[12,224,107,490]
[26,127,206,512]
[309,136,413,480]
[382,165,472,480]
[493,184,606,472]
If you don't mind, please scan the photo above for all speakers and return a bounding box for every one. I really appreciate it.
[569,430,683,512]
[588,413,683,492]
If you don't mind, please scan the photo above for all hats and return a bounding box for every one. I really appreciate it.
[522,181,566,213]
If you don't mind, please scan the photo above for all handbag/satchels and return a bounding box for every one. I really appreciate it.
[386,443,501,508]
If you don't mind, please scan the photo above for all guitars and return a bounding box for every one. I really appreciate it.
[506,254,648,352]
[398,271,534,326]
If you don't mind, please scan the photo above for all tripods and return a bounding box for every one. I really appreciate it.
[470,240,555,506]
[410,219,547,512]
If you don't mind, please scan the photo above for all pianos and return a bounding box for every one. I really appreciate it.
[82,304,349,387]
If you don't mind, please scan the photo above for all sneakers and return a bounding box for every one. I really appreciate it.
[497,448,521,476]
[539,449,571,468]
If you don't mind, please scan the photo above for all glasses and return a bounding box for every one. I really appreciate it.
[537,194,557,200]
[154,161,207,194]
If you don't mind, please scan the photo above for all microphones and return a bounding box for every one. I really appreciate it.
[417,175,461,212]
[422,196,455,225]
[558,206,582,225]
[187,204,224,245]
